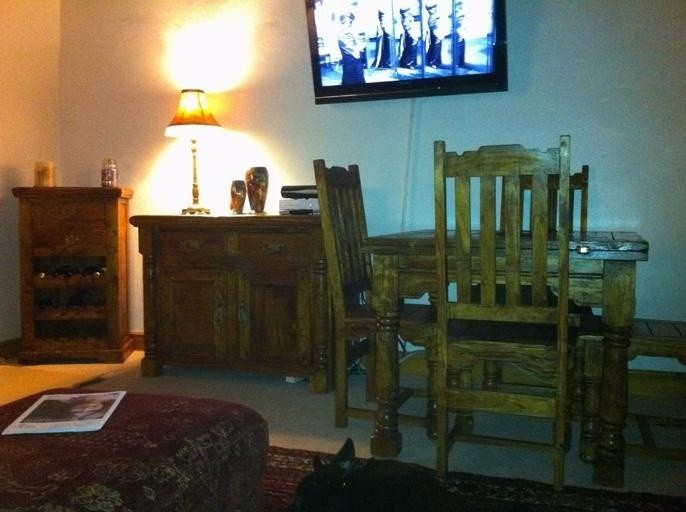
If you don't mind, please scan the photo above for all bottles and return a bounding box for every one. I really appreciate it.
[102,159,118,186]
[93,266,106,278]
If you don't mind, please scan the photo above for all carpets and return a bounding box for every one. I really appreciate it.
[266,444,686,512]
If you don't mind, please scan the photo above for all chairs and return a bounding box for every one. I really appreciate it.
[487,163,590,461]
[572,318,686,468]
[430,135,578,488]
[311,156,457,443]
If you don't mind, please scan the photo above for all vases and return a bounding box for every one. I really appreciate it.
[230,165,268,214]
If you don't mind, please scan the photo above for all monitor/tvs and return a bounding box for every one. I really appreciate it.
[305,0,507,104]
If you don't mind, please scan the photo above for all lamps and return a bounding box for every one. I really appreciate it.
[163,89,220,216]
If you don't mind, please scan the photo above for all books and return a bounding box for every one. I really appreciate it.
[1,389,127,436]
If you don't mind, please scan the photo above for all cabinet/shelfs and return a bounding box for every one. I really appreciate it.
[130,215,330,394]
[10,186,134,364]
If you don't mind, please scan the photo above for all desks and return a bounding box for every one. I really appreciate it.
[355,228,650,496]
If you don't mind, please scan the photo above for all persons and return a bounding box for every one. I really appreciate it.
[66,398,104,421]
[336,0,468,87]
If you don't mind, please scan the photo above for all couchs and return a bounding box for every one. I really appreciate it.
[0,388,269,511]
